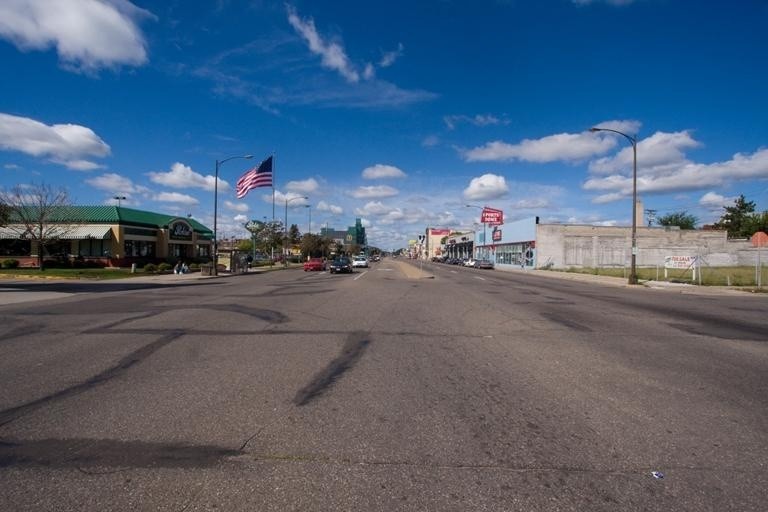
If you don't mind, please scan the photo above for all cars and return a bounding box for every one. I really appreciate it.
[431,256,493,270]
[304,253,380,274]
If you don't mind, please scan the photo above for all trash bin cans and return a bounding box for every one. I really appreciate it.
[200,265,210,276]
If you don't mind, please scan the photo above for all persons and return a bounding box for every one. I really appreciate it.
[182,260,189,274]
[173,260,185,274]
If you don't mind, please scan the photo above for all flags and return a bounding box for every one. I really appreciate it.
[236,156,273,200]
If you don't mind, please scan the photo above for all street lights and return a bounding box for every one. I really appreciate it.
[466,205,486,259]
[590,128,640,286]
[211,154,254,275]
[284,196,308,266]
